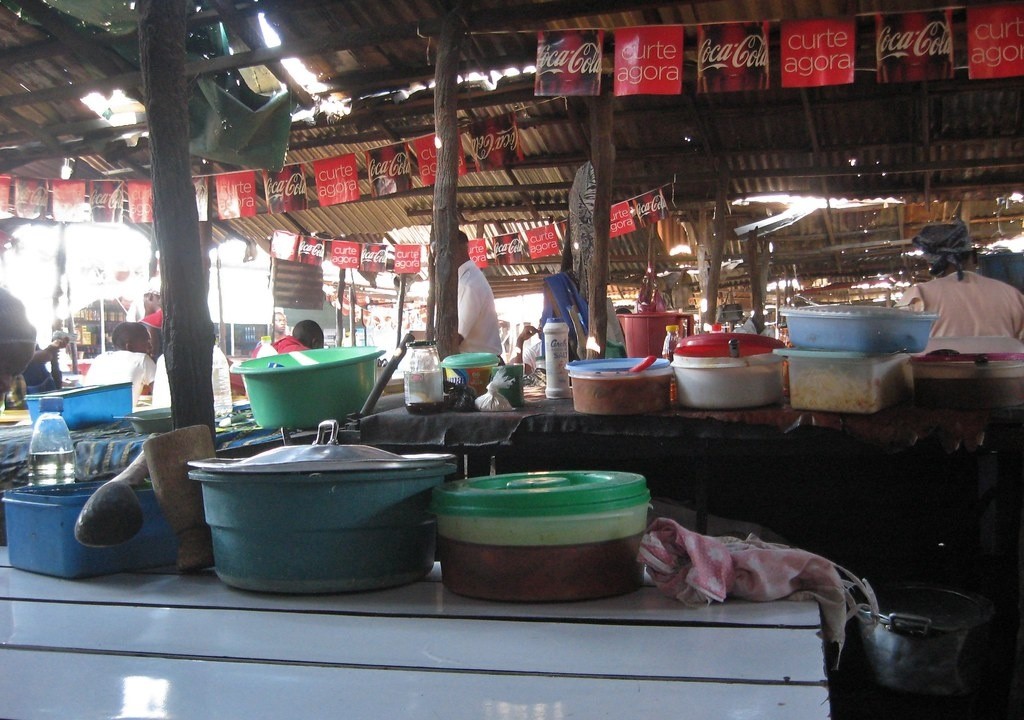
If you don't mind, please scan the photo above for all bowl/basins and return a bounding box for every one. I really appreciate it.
[427,468,652,604]
[910,360,1024,409]
[230,345,387,431]
[186,463,459,593]
[671,351,786,410]
[565,359,673,415]
[126,408,173,433]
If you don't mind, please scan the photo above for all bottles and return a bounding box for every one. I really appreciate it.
[27,396,76,486]
[211,338,233,414]
[404,340,444,414]
[543,318,570,399]
[256,337,278,359]
[662,326,682,402]
[152,354,173,407]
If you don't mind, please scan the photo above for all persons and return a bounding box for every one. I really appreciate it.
[893,224,1024,339]
[273,319,325,353]
[514,317,543,374]
[85,321,157,406]
[135,276,163,362]
[430,229,505,366]
[22,337,70,393]
[275,312,286,341]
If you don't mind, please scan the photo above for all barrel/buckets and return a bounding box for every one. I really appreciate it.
[616,312,692,358]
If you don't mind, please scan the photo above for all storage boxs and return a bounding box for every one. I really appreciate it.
[978,252,1024,295]
[907,358,1024,412]
[772,347,915,416]
[22,381,134,432]
[773,304,940,353]
[3,479,181,582]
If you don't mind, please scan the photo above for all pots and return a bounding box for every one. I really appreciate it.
[853,581,996,697]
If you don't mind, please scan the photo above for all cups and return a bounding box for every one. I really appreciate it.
[491,364,524,407]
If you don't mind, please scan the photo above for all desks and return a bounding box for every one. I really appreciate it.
[0,546,833,720]
[348,386,1024,600]
